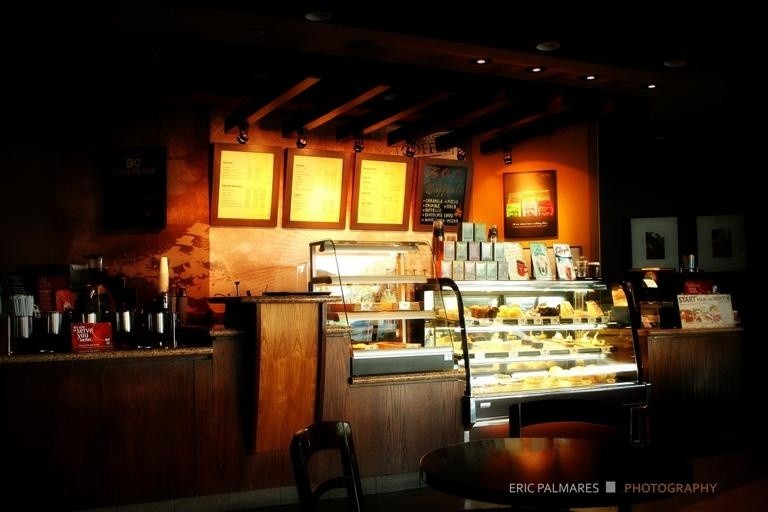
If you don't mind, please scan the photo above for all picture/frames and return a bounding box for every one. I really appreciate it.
[411,157,475,233]
[624,209,754,282]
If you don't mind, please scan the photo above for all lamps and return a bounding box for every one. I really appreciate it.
[237,128,513,167]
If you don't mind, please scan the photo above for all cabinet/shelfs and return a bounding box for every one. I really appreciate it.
[307,239,653,423]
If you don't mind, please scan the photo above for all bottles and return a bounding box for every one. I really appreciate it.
[81,285,96,323]
[430,217,445,279]
[157,291,172,346]
[115,277,131,346]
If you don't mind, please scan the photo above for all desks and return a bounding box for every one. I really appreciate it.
[415,436,698,508]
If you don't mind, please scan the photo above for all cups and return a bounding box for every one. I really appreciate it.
[576,260,600,277]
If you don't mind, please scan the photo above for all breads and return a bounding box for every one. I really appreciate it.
[436,299,615,396]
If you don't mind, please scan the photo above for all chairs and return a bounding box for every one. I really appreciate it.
[508,398,650,450]
[289,421,367,512]
